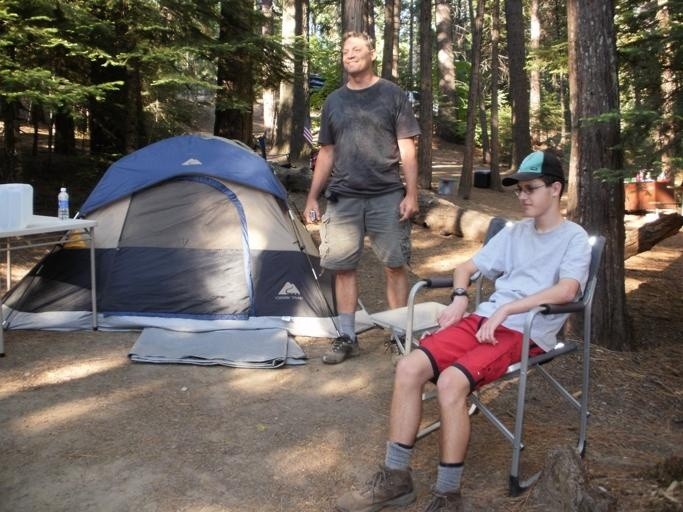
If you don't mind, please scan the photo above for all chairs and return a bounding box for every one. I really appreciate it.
[404,216,607,497]
[0,183,34,215]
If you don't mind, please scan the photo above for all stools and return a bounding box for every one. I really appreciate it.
[370,300,470,355]
[474,172,491,189]
[438,176,458,195]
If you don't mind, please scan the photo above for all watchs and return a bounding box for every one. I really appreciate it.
[450,288,468,302]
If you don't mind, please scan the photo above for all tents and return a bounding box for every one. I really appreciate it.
[1,133,375,340]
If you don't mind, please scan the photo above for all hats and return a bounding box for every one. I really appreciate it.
[501,150,564,187]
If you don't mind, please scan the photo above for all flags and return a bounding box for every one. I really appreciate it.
[309,75,326,90]
[303,126,313,147]
[259,138,267,161]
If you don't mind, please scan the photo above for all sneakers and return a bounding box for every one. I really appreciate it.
[421,483,462,511]
[334,462,416,512]
[322,336,360,363]
[384,337,405,366]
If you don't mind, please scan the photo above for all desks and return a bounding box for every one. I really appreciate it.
[0,215,100,358]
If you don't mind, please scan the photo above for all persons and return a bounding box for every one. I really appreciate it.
[335,150,593,512]
[303,30,421,365]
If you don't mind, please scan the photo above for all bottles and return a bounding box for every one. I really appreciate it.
[56,187,69,222]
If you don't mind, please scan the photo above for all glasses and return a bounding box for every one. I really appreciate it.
[512,184,545,197]
